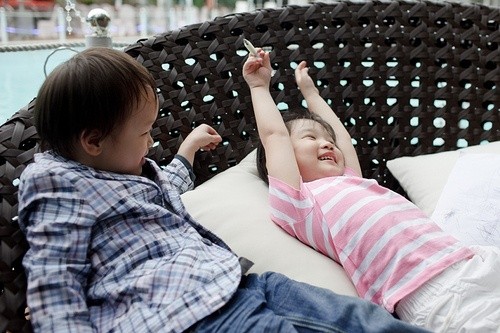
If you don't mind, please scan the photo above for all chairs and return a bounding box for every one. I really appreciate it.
[0,0,500,333]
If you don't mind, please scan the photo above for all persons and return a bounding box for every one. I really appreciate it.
[16,46,435,333]
[242,47,498,333]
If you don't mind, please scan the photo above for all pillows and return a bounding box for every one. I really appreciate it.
[179,147,359,298]
[387,141,500,248]
